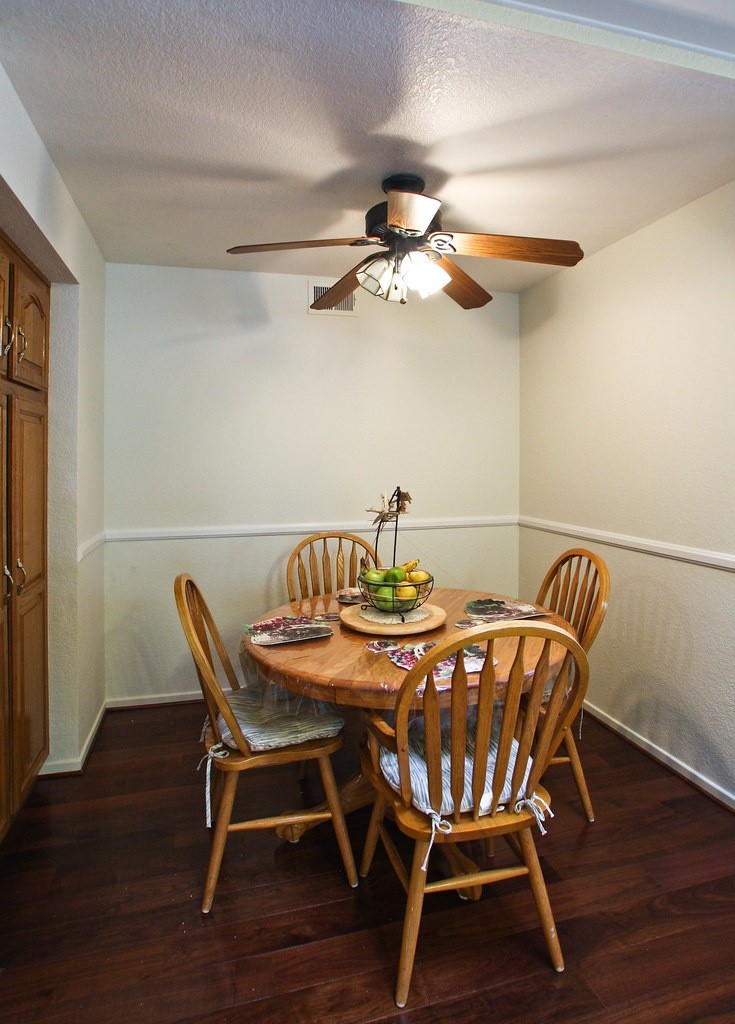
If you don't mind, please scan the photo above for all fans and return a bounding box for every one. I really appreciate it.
[226,173,584,309]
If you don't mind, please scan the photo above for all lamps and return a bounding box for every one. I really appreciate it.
[355,189,452,305]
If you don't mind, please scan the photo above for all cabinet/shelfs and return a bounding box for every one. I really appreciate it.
[0,227,51,842]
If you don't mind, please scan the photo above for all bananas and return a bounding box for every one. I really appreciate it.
[379,559,420,574]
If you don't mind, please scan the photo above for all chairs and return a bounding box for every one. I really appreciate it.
[174,532,610,1007]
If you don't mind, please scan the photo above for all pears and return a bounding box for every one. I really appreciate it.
[395,572,430,601]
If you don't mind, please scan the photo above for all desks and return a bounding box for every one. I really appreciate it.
[243,589,577,901]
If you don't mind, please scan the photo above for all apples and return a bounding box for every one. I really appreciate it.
[362,566,418,610]
